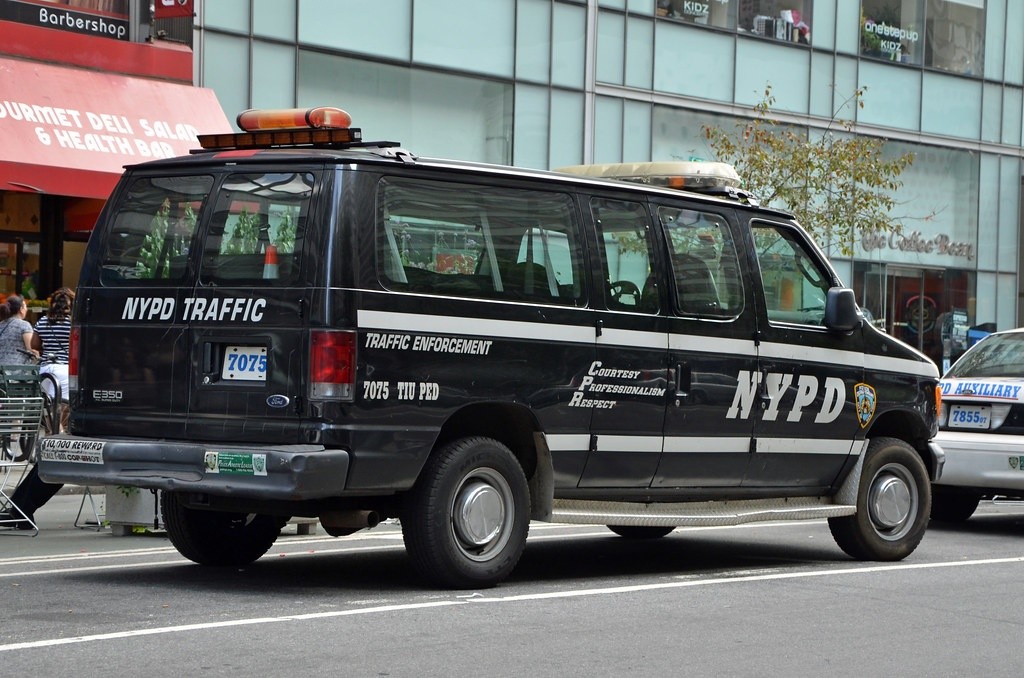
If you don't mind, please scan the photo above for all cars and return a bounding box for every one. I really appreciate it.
[929,327,1024,519]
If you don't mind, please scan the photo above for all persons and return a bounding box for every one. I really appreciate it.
[10,461,64,530]
[0,295,39,456]
[30,288,75,435]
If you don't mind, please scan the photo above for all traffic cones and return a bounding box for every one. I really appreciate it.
[262,245,282,279]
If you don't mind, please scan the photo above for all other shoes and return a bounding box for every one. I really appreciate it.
[0,511,34,530]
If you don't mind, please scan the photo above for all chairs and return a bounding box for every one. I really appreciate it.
[506,262,560,285]
[640,254,721,308]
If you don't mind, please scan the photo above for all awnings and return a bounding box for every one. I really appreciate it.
[1,56,237,200]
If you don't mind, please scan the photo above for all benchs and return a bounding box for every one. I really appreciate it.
[0,364,62,463]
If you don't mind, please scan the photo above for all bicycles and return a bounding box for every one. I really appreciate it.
[2,348,70,463]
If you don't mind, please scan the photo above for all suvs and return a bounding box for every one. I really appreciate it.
[32,103,947,588]
